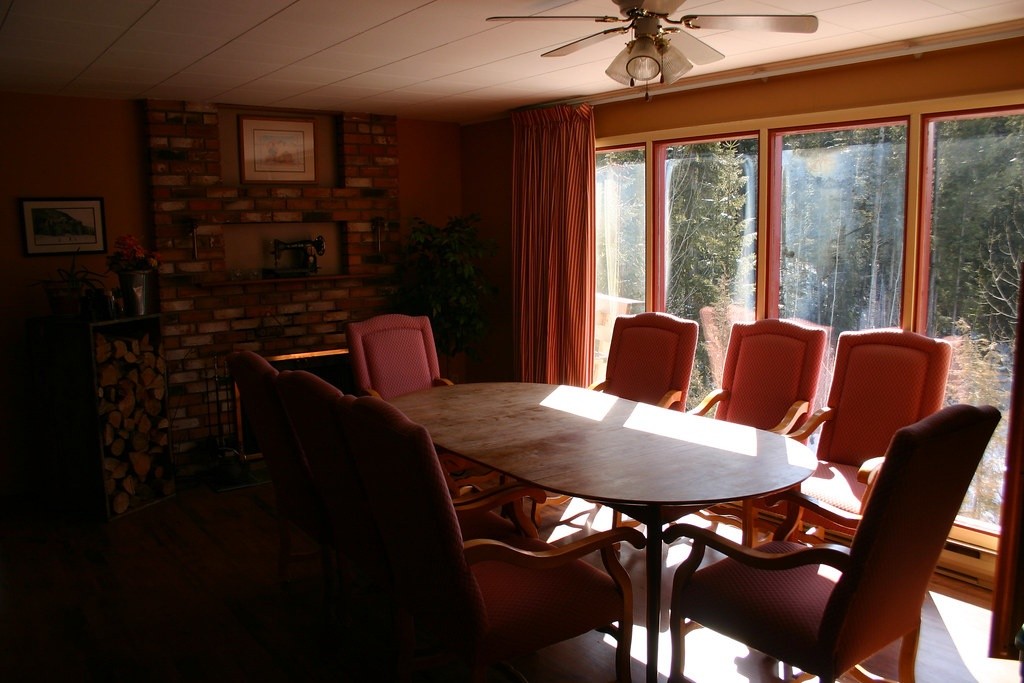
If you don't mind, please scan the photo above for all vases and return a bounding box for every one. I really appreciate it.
[118,271,146,317]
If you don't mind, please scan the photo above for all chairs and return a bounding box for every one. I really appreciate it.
[347,314,488,494]
[667,318,827,535]
[664,404,1002,683]
[339,395,648,683]
[734,326,949,573]
[536,312,699,533]
[227,353,459,583]
[277,370,548,635]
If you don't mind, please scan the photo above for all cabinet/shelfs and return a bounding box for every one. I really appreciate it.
[233,345,349,463]
[25,313,174,523]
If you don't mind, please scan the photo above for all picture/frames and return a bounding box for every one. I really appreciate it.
[21,196,109,256]
[237,116,321,186]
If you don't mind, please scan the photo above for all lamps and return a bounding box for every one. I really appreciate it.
[626,18,670,102]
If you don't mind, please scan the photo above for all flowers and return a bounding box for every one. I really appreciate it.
[109,231,161,272]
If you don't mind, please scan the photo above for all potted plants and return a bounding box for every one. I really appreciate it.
[39,262,103,318]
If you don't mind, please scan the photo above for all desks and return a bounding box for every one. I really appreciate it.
[387,381,817,683]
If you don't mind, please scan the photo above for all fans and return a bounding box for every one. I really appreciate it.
[486,0,820,68]
